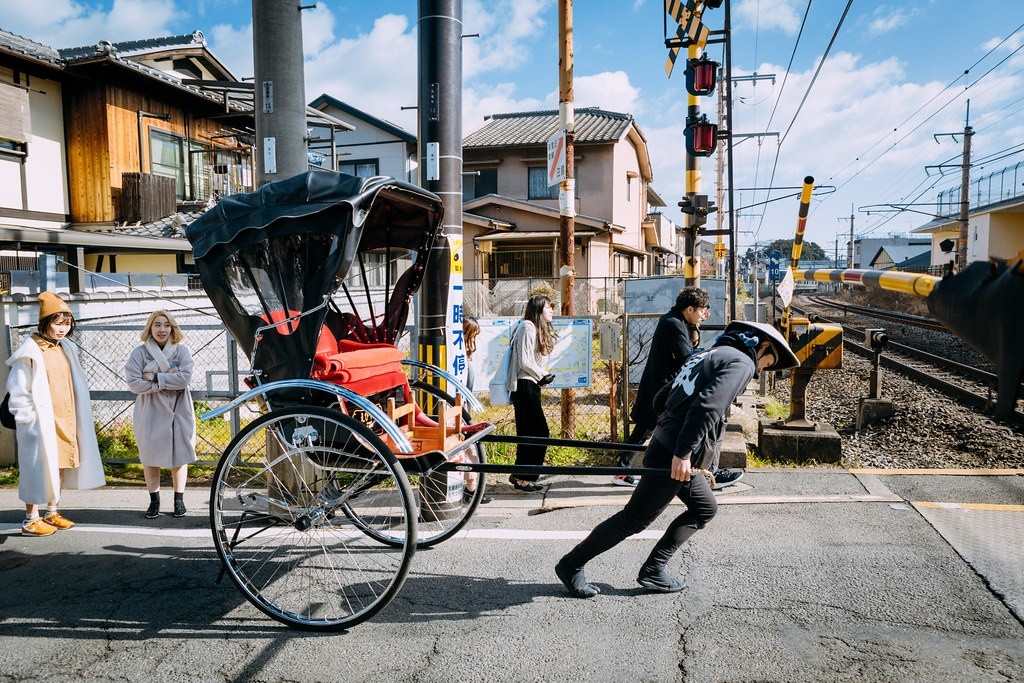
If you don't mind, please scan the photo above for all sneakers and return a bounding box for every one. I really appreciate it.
[146,500,161,518]
[43,511,75,529]
[710,468,744,489]
[173,502,186,516]
[613,475,638,486]
[22,517,57,536]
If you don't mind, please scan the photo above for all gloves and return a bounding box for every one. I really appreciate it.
[538,374,555,386]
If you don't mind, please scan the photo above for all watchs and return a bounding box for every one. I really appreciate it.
[149,374,154,381]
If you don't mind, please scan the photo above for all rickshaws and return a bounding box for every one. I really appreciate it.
[184,166,693,632]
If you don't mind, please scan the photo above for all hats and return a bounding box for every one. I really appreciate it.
[37,291,73,321]
[725,320,800,371]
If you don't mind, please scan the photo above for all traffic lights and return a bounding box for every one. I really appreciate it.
[687,54,720,95]
[685,119,718,159]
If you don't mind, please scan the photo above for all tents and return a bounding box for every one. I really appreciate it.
[184,168,444,387]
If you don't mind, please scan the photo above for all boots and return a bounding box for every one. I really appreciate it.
[637,547,686,591]
[554,545,600,597]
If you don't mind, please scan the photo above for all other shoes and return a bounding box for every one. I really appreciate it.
[509,475,543,491]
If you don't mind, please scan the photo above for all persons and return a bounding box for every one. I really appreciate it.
[125,309,197,518]
[554,320,803,597]
[5,291,107,537]
[611,287,745,490]
[456,314,495,504]
[505,294,563,492]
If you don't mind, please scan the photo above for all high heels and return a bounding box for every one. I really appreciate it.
[463,486,494,503]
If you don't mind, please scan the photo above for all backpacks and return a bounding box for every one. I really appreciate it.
[489,320,537,407]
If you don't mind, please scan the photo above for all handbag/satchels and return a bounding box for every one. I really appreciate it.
[0,391,16,429]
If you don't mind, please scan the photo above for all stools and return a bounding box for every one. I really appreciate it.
[386,390,462,456]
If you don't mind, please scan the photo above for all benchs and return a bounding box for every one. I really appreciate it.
[257,310,419,432]
